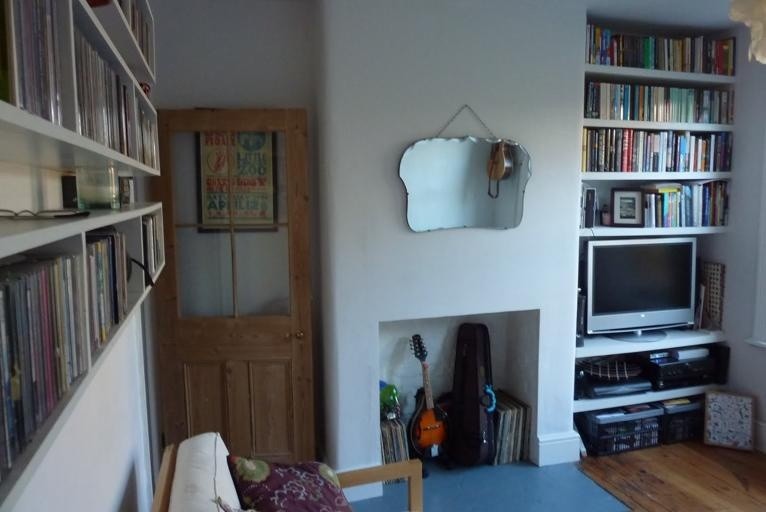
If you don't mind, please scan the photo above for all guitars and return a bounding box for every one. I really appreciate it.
[487,143,513,199]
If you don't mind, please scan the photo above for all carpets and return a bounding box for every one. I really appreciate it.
[347,459,633,511]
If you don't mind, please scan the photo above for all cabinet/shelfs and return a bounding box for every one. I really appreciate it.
[89,0,155,84]
[0,0,160,176]
[580,63,736,228]
[574,330,730,415]
[0,202,165,511]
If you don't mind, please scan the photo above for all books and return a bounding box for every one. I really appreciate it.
[584,80,734,124]
[1,0,64,128]
[143,211,164,288]
[582,127,732,173]
[489,389,531,466]
[380,409,411,485]
[641,178,730,228]
[585,23,735,78]
[121,0,152,70]
[1,251,89,472]
[86,227,127,362]
[582,182,597,227]
[73,28,132,156]
[135,96,158,170]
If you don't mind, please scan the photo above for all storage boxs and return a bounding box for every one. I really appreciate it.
[659,399,705,444]
[583,402,663,456]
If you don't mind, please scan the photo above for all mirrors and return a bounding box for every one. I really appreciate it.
[398,134,531,232]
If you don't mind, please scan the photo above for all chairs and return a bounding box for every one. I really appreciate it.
[149,443,422,511]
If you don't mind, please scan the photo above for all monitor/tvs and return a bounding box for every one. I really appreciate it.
[588,237,696,342]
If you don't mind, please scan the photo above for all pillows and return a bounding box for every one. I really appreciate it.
[227,454,350,511]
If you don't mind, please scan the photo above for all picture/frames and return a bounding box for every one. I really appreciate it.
[193,107,279,233]
[610,186,644,227]
